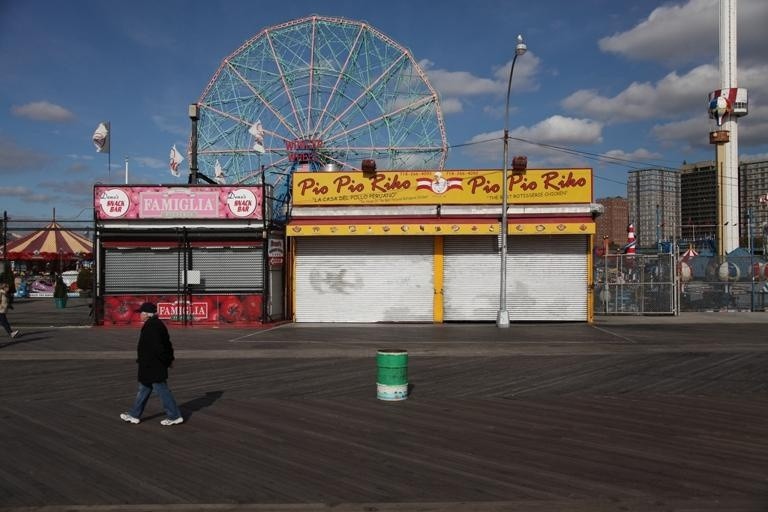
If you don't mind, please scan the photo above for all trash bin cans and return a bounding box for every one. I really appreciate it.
[376,348,409,401]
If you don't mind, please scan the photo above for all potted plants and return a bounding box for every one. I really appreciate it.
[53,276,68,308]
[77,265,93,299]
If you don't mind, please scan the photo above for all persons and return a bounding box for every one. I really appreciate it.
[14,274,23,292]
[0,281,20,339]
[118,301,184,427]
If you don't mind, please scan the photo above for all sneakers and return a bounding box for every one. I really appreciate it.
[160,416,184,426]
[121,414,140,424]
[11,331,19,338]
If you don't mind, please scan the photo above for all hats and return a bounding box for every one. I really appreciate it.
[134,303,157,313]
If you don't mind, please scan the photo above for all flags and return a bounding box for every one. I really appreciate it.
[91,120,109,154]
[247,118,265,155]
[213,159,228,187]
[168,143,185,178]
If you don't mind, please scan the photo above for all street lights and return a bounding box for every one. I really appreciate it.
[494,43,529,326]
